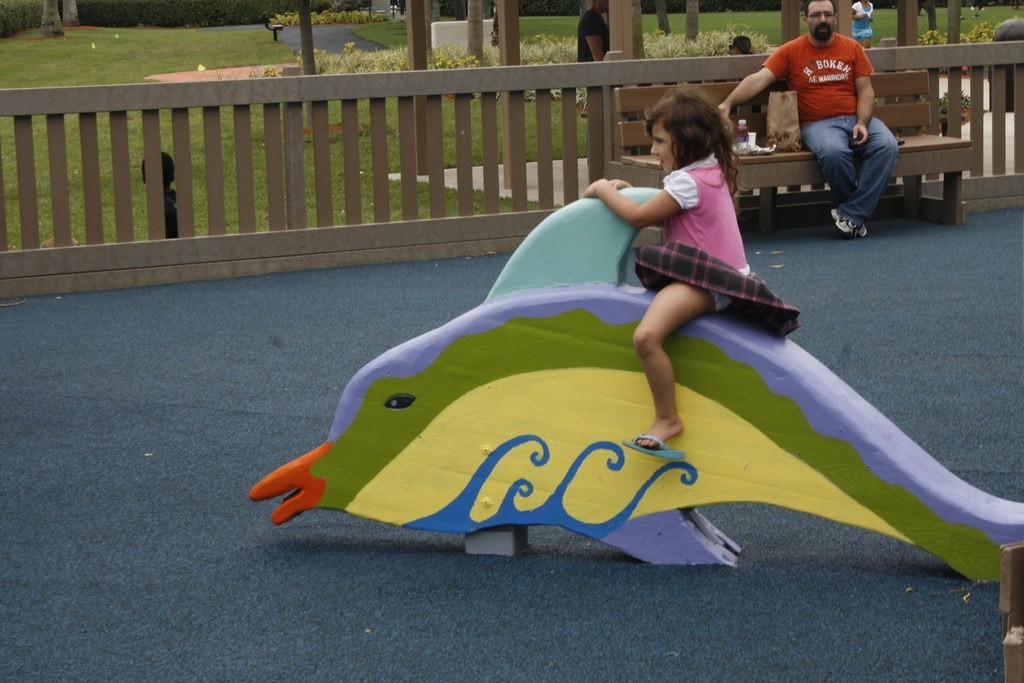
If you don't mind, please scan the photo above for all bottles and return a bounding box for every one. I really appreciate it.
[737,120,748,142]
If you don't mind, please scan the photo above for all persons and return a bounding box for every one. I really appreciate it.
[396,0,407,22]
[851,0,873,49]
[141,151,179,239]
[577,0,609,160]
[579,88,802,463]
[727,36,752,55]
[490,4,499,47]
[716,0,898,241]
[970,0,986,12]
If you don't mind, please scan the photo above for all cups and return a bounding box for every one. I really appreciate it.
[748,132,756,149]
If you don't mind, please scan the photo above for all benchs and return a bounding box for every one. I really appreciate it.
[602,69,974,249]
[263,12,283,41]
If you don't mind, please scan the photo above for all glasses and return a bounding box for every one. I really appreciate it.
[806,11,835,19]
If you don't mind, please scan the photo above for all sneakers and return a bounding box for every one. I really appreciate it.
[831,207,867,240]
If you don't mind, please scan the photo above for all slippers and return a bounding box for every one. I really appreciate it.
[623,434,684,460]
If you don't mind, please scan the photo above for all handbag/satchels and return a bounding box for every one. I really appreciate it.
[766,90,802,153]
